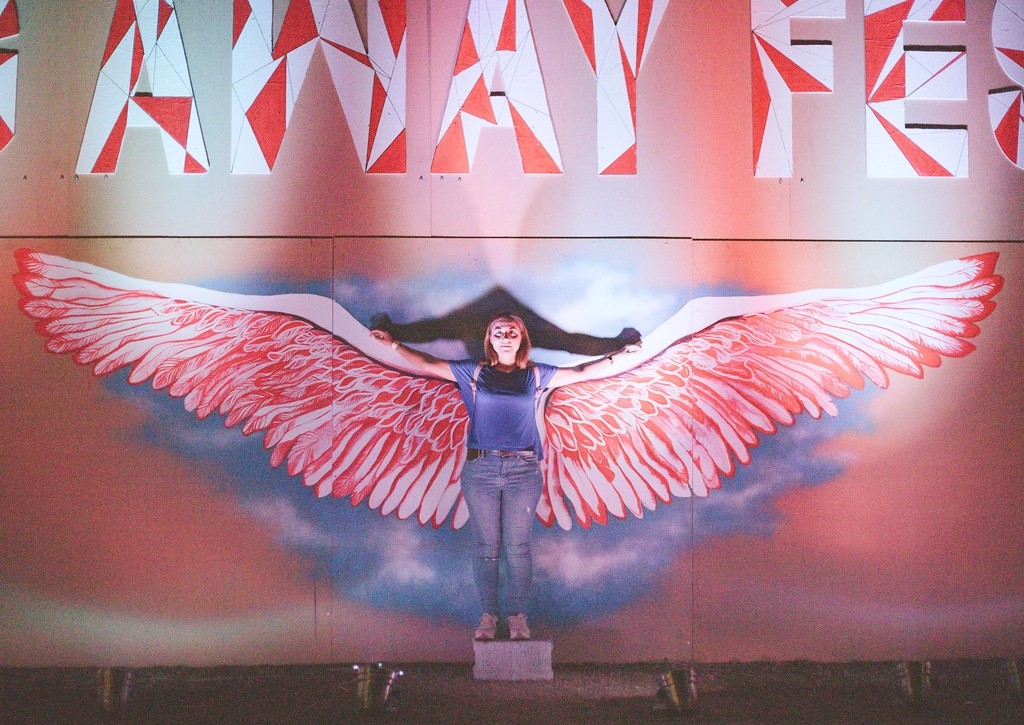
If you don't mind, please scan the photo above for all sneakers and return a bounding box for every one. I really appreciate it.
[507,613,530,639]
[474,611,498,640]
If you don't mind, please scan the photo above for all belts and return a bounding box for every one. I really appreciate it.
[468,448,535,458]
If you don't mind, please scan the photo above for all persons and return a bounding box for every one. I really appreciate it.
[368,313,643,642]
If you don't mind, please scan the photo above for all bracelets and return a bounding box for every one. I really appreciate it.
[392,340,401,351]
[605,353,614,365]
